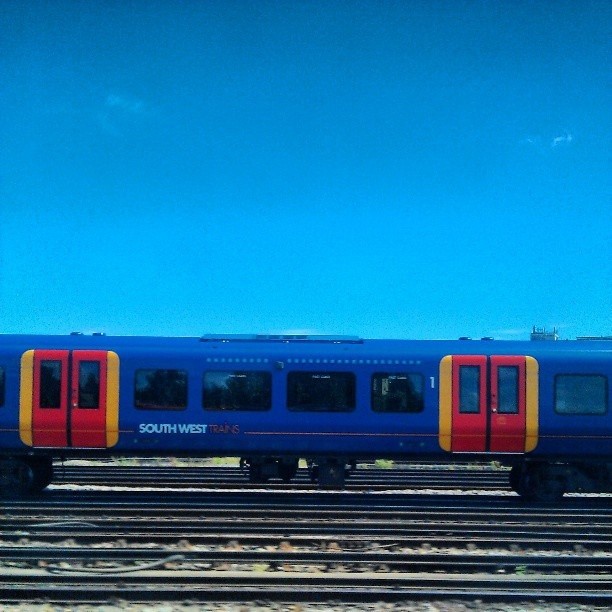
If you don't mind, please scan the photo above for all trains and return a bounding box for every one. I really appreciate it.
[1,335,611,500]
